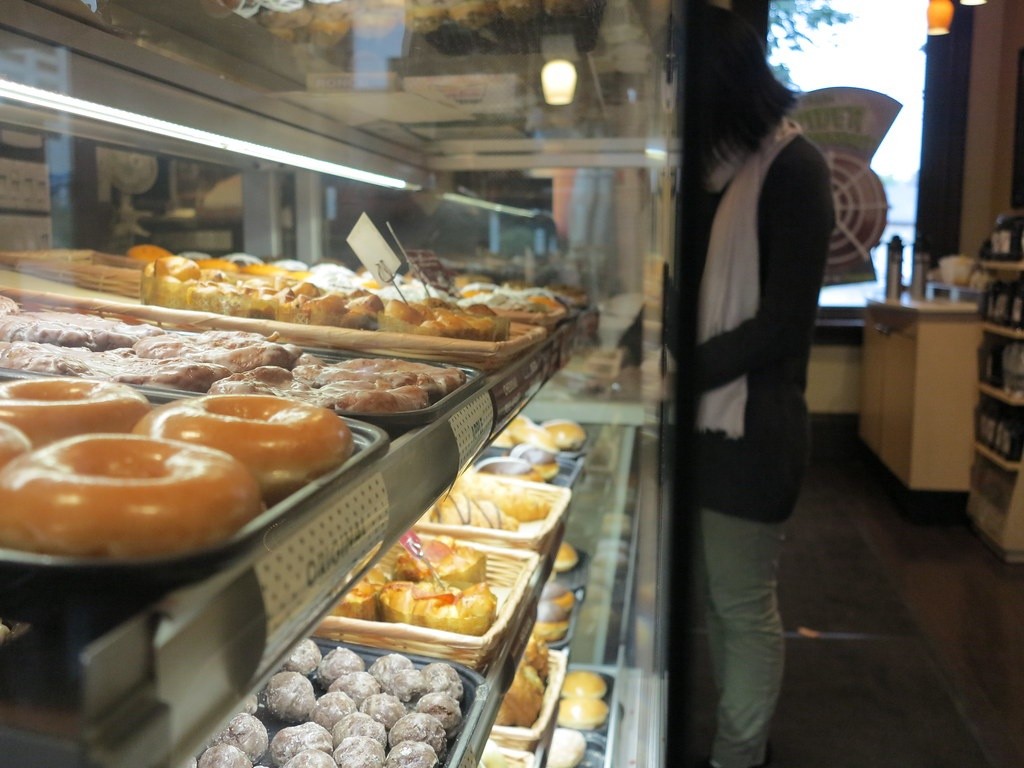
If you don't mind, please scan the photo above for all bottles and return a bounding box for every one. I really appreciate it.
[978,215,1024,461]
[910,235,930,301]
[886,236,902,299]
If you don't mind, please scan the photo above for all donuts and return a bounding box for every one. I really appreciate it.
[0,376,362,562]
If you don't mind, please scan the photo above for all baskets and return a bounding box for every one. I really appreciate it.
[312,534,541,670]
[3,246,567,374]
[477,648,569,767]
[412,471,573,562]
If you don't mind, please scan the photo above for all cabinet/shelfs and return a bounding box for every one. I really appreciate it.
[858,294,983,500]
[0,25,671,768]
[967,258,1024,564]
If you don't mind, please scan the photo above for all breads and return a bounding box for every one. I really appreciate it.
[0,244,585,413]
[327,414,613,768]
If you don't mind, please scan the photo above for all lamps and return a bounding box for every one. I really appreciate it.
[927,0,955,36]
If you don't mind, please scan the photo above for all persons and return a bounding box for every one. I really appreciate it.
[608,1,839,768]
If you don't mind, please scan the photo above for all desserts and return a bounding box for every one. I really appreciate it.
[182,639,464,768]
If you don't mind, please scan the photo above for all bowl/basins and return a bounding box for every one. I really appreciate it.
[938,256,974,286]
